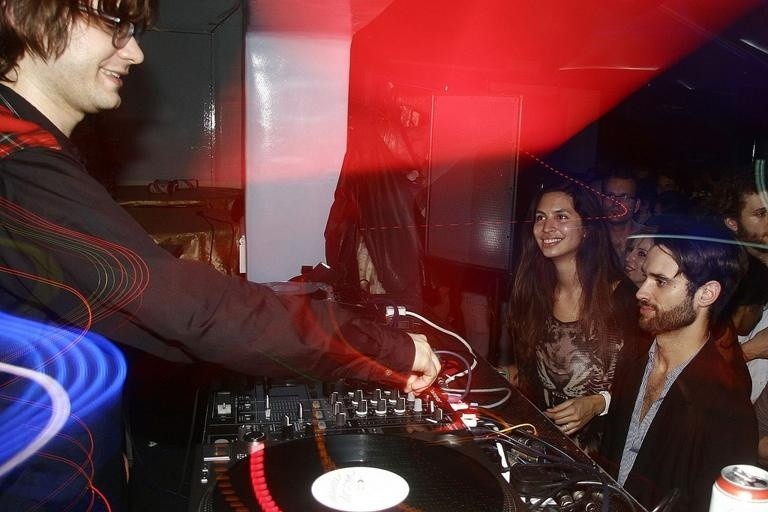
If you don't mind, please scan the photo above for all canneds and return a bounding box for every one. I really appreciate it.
[708,464,768,512]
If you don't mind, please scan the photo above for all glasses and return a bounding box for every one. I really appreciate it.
[63,0,146,49]
[605,192,636,202]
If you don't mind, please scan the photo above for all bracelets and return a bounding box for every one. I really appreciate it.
[499,365,511,384]
[597,390,611,417]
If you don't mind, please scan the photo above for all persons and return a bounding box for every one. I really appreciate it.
[0,0,442,512]
[492,182,639,460]
[753,384,768,471]
[594,218,758,512]
